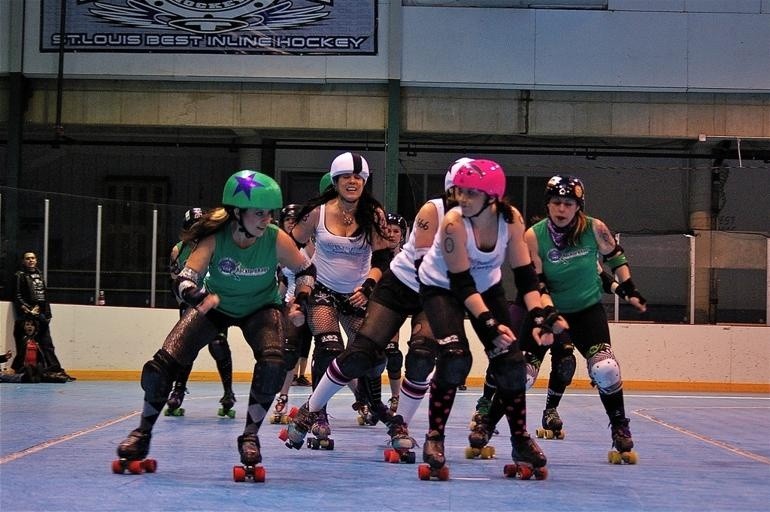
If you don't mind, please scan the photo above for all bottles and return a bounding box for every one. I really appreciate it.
[98,291,105,305]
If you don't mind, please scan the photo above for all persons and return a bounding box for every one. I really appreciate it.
[0,349,37,385]
[14,250,77,382]
[109,153,645,485]
[10,314,50,382]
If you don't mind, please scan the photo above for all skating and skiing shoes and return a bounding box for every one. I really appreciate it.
[112,427,158,475]
[234,432,265,482]
[503,434,548,479]
[270,396,416,480]
[469,397,492,431]
[536,408,565,439]
[608,419,637,463]
[466,414,495,459]
[218,392,235,417]
[164,389,185,416]
[418,433,448,480]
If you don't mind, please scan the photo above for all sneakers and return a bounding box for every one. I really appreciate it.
[35,365,42,382]
[21,366,33,382]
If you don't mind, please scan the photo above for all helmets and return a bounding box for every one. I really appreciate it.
[183,207,212,232]
[386,213,407,239]
[544,173,586,213]
[330,151,369,188]
[279,204,303,228]
[453,159,506,203]
[222,168,283,210]
[444,157,471,193]
